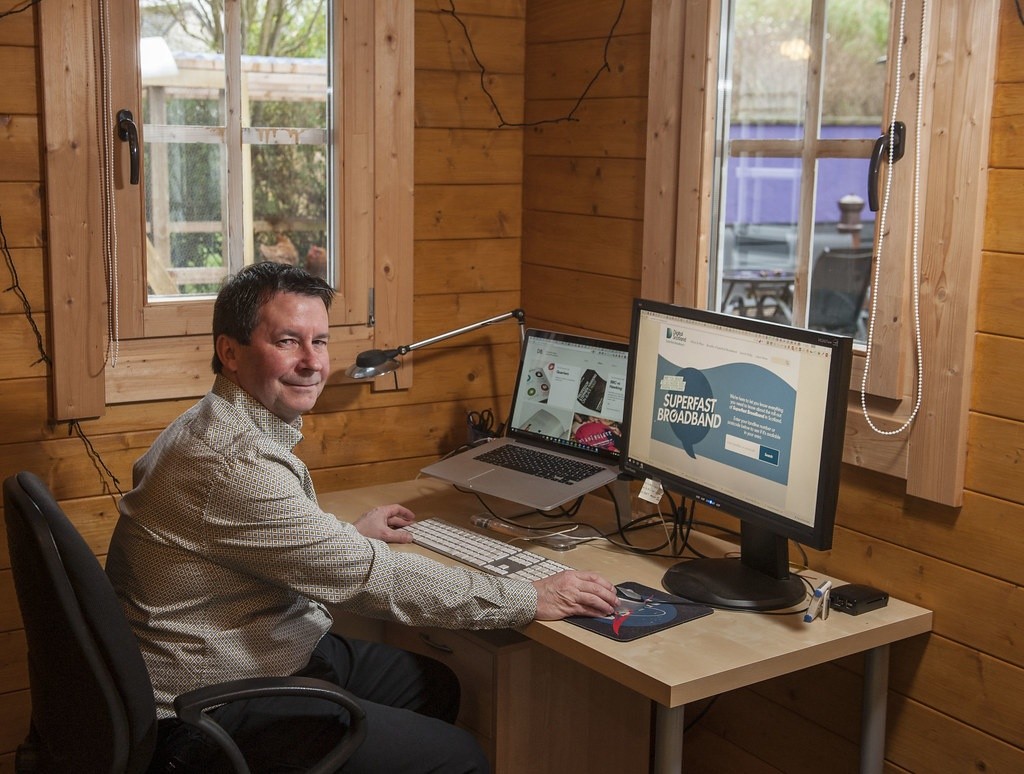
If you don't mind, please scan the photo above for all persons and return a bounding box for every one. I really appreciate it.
[104,262,620,774]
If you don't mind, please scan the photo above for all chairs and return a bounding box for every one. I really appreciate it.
[3,472,368,774]
[755,246,873,337]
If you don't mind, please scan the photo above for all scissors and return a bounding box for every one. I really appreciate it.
[466,408,494,434]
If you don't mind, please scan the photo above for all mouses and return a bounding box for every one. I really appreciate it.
[611,585,646,613]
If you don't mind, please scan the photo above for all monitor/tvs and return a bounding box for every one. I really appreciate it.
[620,295,855,610]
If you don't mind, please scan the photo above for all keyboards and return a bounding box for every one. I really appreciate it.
[397,516,576,583]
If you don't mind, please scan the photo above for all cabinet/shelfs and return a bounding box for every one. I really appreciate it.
[387,621,651,774]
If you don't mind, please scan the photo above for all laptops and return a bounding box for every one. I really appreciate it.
[419,327,630,511]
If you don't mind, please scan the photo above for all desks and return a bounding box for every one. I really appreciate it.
[316,476,933,774]
[721,268,799,320]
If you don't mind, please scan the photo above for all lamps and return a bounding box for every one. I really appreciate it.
[344,308,527,380]
[139,36,178,80]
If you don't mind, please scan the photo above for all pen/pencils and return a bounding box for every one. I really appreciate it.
[483,406,511,437]
